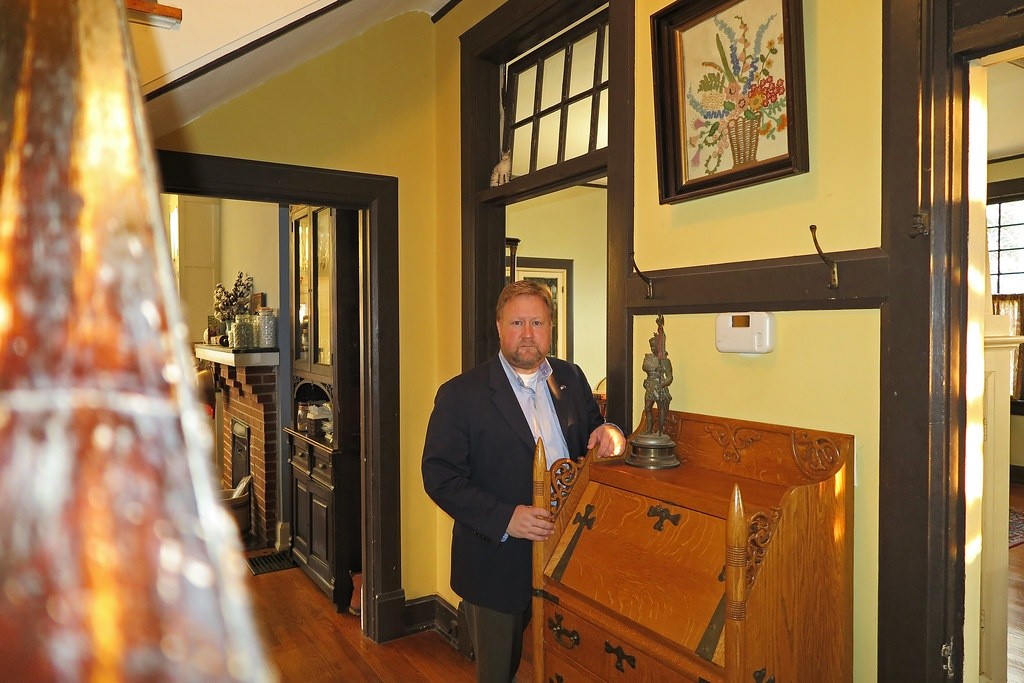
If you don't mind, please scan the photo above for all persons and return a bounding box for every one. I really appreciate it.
[421,280,627,683]
[642,332,673,435]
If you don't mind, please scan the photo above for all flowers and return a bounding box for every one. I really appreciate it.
[211,272,255,324]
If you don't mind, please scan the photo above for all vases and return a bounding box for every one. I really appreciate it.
[224,319,235,336]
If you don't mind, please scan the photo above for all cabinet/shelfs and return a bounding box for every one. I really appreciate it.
[533,409,854,683]
[283,204,360,613]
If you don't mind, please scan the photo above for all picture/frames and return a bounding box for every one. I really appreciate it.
[650,0,810,205]
[506,256,574,363]
